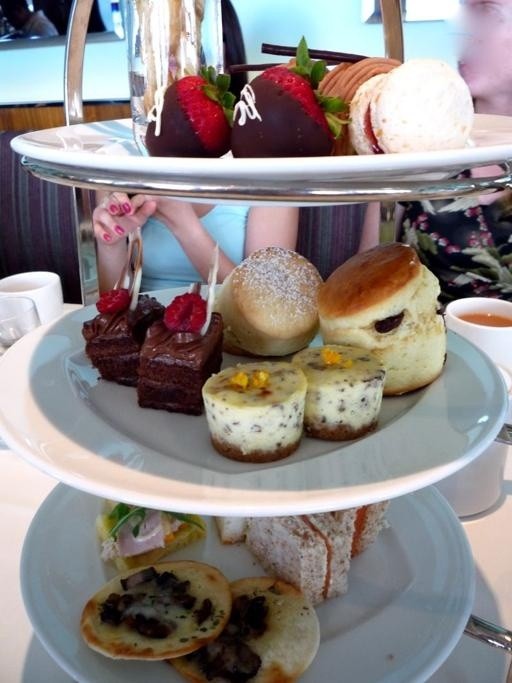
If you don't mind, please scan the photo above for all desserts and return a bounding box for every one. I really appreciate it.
[81,499,387,683]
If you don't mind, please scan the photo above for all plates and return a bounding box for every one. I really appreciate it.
[6,108,512,209]
[16,481,480,682]
[0,282,512,523]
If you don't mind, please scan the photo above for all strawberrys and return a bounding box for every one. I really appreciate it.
[230,36,354,158]
[144,63,237,157]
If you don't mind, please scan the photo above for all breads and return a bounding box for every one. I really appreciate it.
[316,57,475,155]
[318,242,448,395]
[213,245,324,361]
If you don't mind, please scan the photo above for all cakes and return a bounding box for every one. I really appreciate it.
[137,242,225,415]
[201,360,308,462]
[291,344,387,441]
[81,225,163,387]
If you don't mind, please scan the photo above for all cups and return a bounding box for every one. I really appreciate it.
[444,295,512,370]
[117,0,225,145]
[0,269,65,348]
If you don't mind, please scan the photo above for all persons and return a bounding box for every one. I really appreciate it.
[90,181,299,306]
[0,0,61,41]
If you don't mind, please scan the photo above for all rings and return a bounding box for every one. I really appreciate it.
[99,195,110,208]
